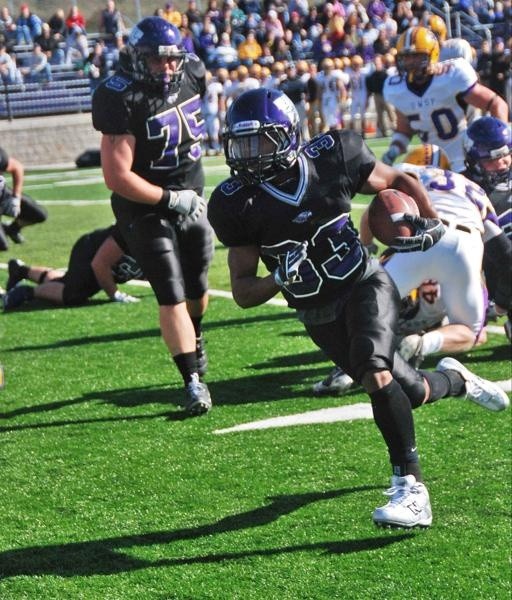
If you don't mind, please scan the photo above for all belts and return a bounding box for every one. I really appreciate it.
[440,218,471,233]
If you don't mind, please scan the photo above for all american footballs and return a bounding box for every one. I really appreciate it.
[367,189,420,243]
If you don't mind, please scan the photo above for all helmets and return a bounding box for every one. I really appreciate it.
[462,116,512,191]
[418,15,448,43]
[400,143,450,171]
[205,46,397,83]
[126,17,187,95]
[223,86,301,185]
[395,26,440,83]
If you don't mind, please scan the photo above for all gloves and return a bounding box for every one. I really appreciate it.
[274,240,308,286]
[111,289,141,303]
[390,212,445,253]
[168,190,206,222]
[1,197,22,217]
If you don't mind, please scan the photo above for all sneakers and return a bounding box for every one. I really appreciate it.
[185,372,212,417]
[435,357,510,412]
[196,332,207,376]
[6,258,29,290]
[397,330,426,371]
[371,474,432,531]
[4,286,33,313]
[5,225,24,244]
[312,365,355,398]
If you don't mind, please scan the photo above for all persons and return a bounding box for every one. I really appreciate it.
[1,0,512,400]
[90,15,225,416]
[207,88,512,528]
